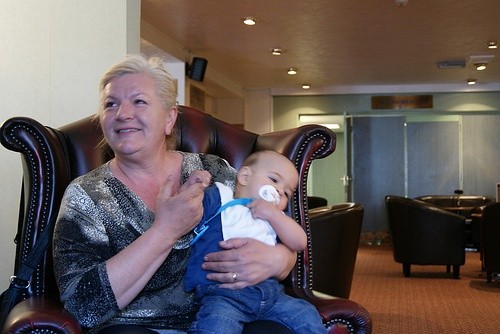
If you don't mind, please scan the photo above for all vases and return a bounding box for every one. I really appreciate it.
[456,198,461,207]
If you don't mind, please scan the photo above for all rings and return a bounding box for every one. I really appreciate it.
[232,273,238,282]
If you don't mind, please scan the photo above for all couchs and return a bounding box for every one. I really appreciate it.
[385,195,465,279]
[308,201,366,300]
[480,202,500,284]
[0,106,374,334]
[414,191,497,253]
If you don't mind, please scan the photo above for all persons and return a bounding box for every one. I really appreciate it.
[176,150,330,334]
[52,55,299,334]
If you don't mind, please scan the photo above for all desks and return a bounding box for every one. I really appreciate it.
[440,206,475,215]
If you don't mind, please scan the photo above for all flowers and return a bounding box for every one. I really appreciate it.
[454,190,463,198]
